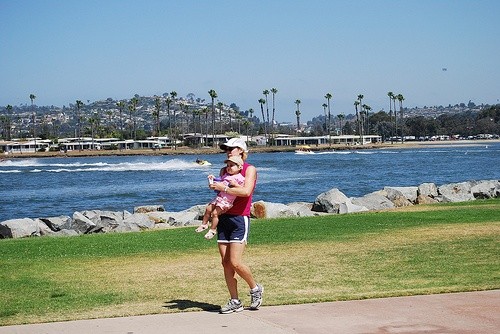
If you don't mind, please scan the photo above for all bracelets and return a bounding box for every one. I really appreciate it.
[224,186,229,194]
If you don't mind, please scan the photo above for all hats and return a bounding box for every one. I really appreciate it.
[224,156,244,165]
[220,138,248,154]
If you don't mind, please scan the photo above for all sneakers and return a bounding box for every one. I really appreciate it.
[247,283,265,309]
[219,299,245,313]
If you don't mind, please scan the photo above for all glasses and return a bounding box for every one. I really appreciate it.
[224,146,233,151]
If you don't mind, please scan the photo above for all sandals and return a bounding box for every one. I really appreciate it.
[195,224,209,233]
[204,226,218,240]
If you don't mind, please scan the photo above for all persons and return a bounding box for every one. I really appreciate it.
[210,138,264,314]
[195,156,245,239]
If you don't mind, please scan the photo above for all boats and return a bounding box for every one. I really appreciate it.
[196,157,205,165]
[295,148,311,153]
